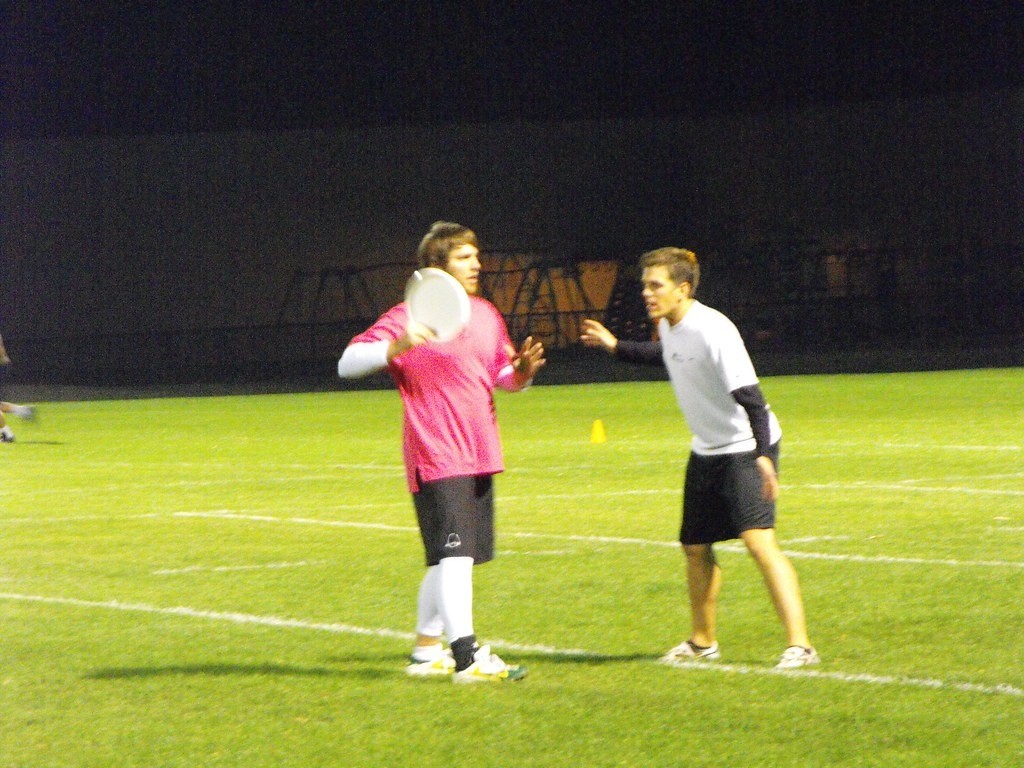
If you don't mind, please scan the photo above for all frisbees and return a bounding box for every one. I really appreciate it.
[404,264,472,344]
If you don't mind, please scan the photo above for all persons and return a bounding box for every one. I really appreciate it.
[585,246,819,668]
[337,220,547,681]
[0,334,39,442]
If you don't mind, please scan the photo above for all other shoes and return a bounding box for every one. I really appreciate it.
[0,431,14,443]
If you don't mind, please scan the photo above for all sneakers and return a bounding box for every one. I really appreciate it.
[405,648,457,674]
[658,641,720,660]
[452,645,528,686]
[775,647,821,668]
